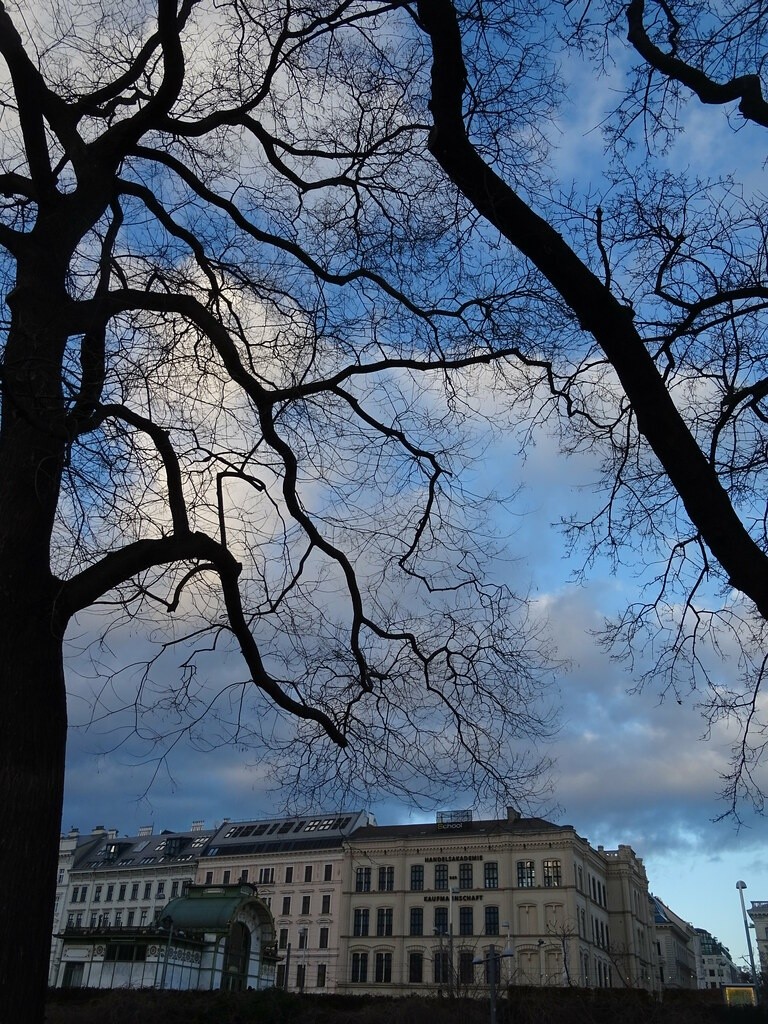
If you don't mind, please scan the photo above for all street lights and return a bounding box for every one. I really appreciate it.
[449,887,461,984]
[298,926,308,993]
[503,923,513,986]
[735,879,762,1005]
[433,927,445,985]
